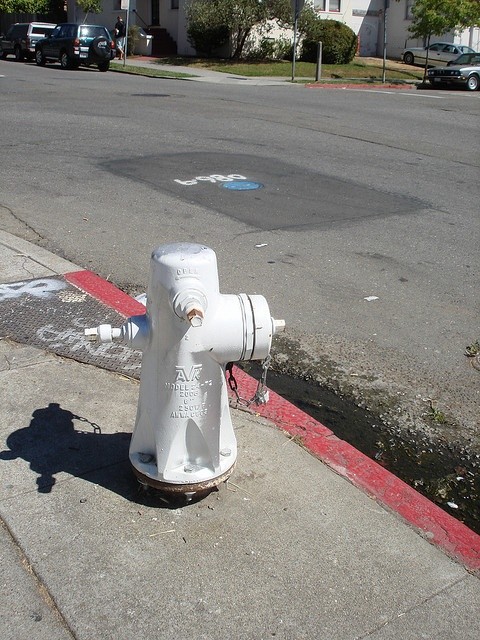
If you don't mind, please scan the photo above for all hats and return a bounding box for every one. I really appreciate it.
[427,52,480,91]
[400,42,476,67]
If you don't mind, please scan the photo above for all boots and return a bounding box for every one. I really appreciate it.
[35,22,116,72]
[0,22,57,62]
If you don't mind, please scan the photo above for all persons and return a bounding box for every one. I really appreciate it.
[114,15,126,60]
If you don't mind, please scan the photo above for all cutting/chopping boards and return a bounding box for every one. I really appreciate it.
[82,242,288,499]
